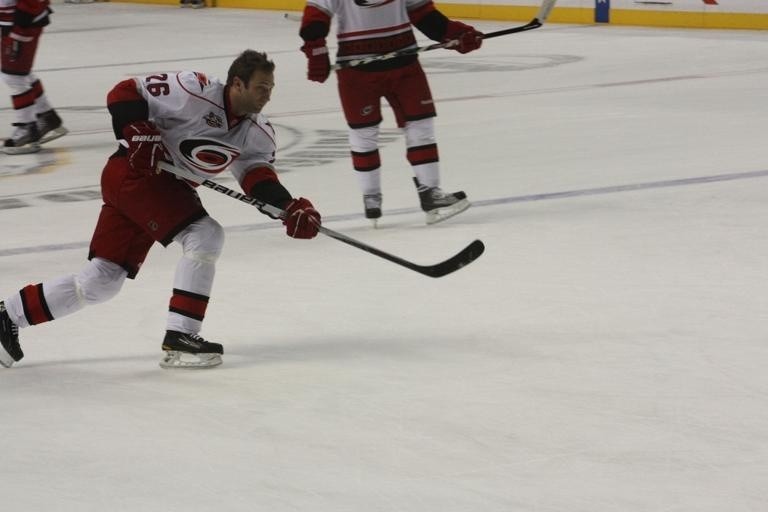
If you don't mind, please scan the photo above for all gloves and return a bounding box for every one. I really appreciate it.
[123,120,171,175]
[302,40,329,82]
[443,22,483,54]
[283,197,320,238]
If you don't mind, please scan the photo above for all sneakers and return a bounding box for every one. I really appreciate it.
[5,111,63,146]
[0,301,24,361]
[162,330,223,352]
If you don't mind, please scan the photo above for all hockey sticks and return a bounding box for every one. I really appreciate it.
[158,163,485,277]
[330,0,554,72]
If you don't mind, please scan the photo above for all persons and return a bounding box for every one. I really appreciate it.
[0,48,321,361]
[0,0,63,148]
[299,0,485,220]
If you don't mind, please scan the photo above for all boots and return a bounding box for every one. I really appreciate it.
[364,193,381,217]
[413,177,465,211]
